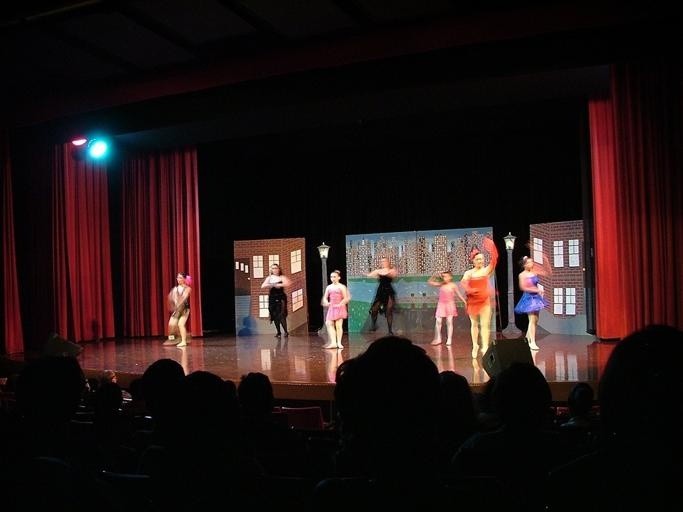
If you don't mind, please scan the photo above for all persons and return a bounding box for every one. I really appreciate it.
[164,273,191,346]
[362,257,398,335]
[320,269,351,349]
[460,237,499,357]
[260,264,292,337]
[433,345,454,374]
[323,348,344,384]
[0,322,683,511]
[513,251,552,349]
[427,269,466,346]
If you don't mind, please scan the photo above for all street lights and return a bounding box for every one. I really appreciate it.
[501,232,523,334]
[317,240,332,326]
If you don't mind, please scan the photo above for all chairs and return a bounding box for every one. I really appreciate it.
[0,388,681,511]
